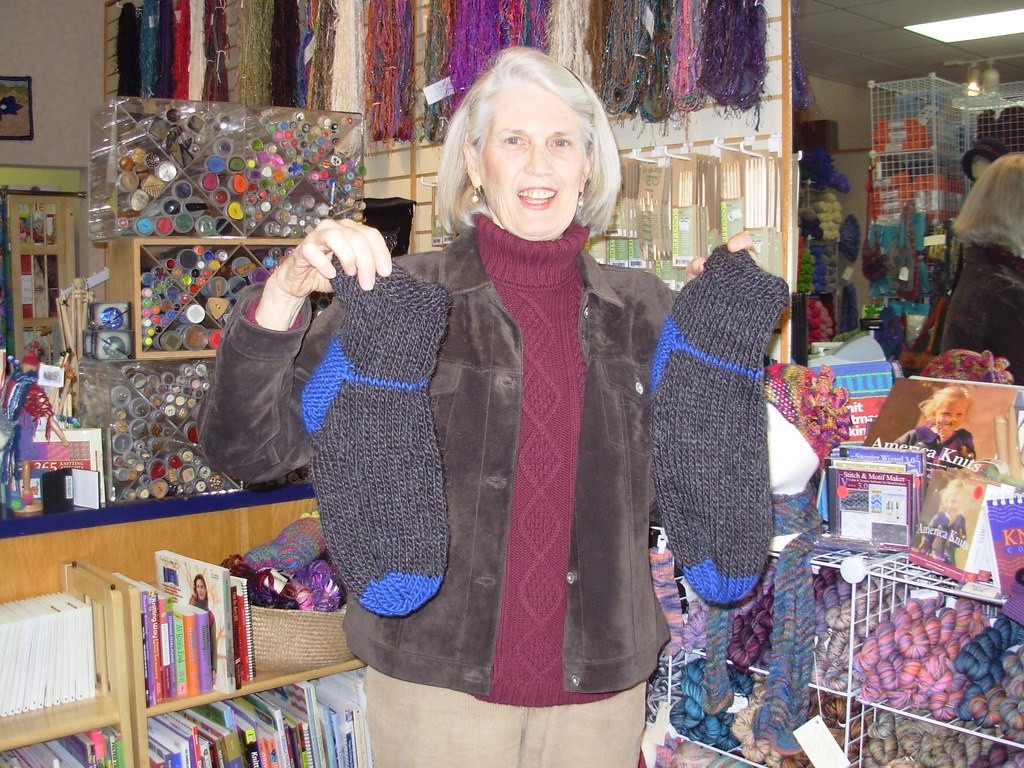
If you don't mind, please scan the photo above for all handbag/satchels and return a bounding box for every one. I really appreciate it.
[895,208,919,301]
[887,210,914,292]
[861,167,883,282]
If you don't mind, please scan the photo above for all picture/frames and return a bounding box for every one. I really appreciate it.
[0,76,33,140]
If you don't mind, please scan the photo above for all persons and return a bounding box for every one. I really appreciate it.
[892,388,977,473]
[190,574,218,685]
[942,151,1024,388]
[199,48,767,767]
[917,479,967,567]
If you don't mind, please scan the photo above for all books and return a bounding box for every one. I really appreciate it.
[824,444,1024,596]
[0,546,372,768]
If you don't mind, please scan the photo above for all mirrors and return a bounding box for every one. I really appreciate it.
[782,0,1024,390]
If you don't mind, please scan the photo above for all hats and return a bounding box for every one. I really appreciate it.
[920,346,1014,387]
[763,362,853,471]
[962,138,1008,182]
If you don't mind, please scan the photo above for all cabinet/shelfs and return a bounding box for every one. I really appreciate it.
[867,72,1024,305]
[0,98,371,768]
[638,530,1024,768]
